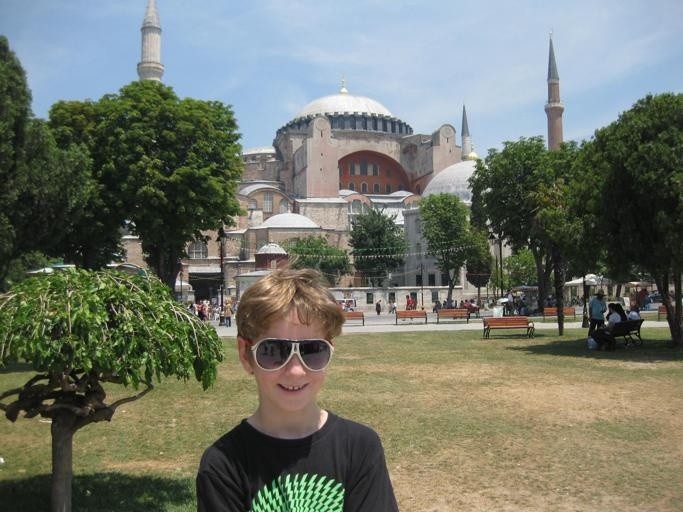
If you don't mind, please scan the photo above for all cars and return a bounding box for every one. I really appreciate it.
[646,290,672,304]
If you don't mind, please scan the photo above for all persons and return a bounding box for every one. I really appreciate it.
[338,293,498,321]
[182,294,240,327]
[196,261,400,511]
[503,285,682,353]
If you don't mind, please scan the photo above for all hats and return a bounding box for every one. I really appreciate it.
[594,289,607,297]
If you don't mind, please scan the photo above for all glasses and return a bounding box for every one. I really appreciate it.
[247,337,334,374]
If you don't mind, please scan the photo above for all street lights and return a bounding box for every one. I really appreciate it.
[215,223,231,328]
[178,272,185,306]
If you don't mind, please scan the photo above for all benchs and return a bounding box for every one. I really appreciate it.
[657,306,675,322]
[436,309,469,325]
[542,308,576,321]
[479,316,535,340]
[342,311,365,328]
[396,310,427,325]
[590,319,644,349]
